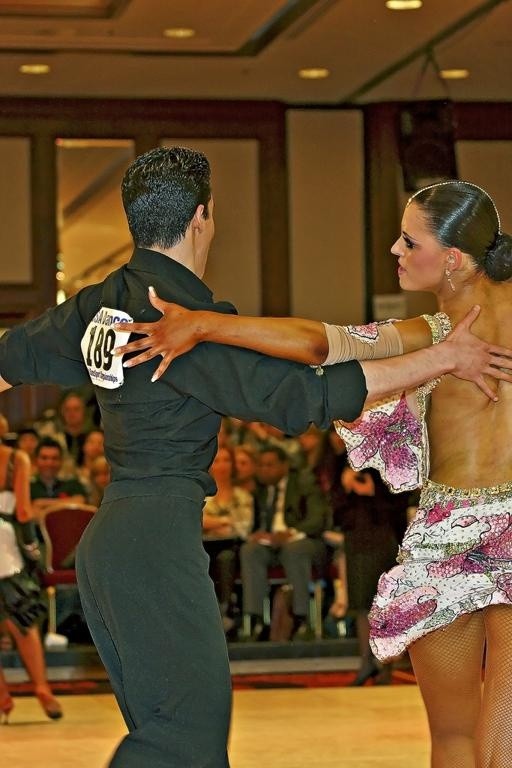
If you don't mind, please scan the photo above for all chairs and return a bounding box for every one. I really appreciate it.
[347,647,397,688]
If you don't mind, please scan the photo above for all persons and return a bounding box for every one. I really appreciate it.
[201,410,348,643]
[334,443,419,685]
[0,143,510,764]
[1,414,64,720]
[103,170,512,765]
[0,388,109,638]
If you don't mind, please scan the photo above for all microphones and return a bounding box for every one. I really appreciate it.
[233,565,341,642]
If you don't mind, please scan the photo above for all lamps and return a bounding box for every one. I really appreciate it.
[38,502,98,644]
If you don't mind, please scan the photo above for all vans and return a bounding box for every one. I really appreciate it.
[0,687,64,724]
